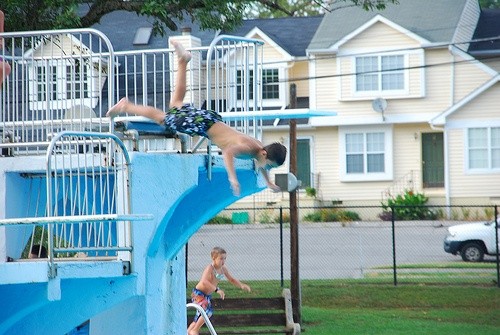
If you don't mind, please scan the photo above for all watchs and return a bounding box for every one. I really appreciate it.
[215,287,220,292]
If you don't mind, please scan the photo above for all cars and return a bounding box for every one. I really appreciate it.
[443,213,500,263]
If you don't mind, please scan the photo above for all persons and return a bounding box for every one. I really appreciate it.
[28,246,48,258]
[0,11,11,89]
[106,41,286,195]
[186,247,251,335]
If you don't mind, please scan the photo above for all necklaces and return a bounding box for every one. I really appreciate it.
[211,265,224,278]
